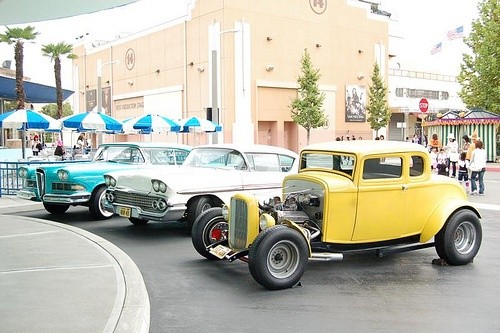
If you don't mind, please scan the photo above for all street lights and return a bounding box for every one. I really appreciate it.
[96,59,119,114]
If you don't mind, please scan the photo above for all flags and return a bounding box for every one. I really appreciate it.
[432,42,441,54]
[448,26,463,40]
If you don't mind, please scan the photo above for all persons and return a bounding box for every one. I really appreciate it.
[31,135,41,156]
[75,133,87,154]
[426,132,486,196]
[406,133,428,148]
[375,135,384,140]
[346,88,365,115]
[336,135,362,165]
[54,145,65,156]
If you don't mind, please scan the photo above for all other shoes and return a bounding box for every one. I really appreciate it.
[479,192,484,195]
[471,190,478,195]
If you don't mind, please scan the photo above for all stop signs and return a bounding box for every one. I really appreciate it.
[419,98,429,112]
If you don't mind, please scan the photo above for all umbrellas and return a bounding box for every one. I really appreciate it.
[0,109,61,159]
[45,111,223,146]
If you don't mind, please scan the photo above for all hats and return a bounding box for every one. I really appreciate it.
[447,133,455,139]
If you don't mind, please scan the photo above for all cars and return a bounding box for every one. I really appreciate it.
[192,140,483,291]
[16,142,194,221]
[102,142,299,236]
